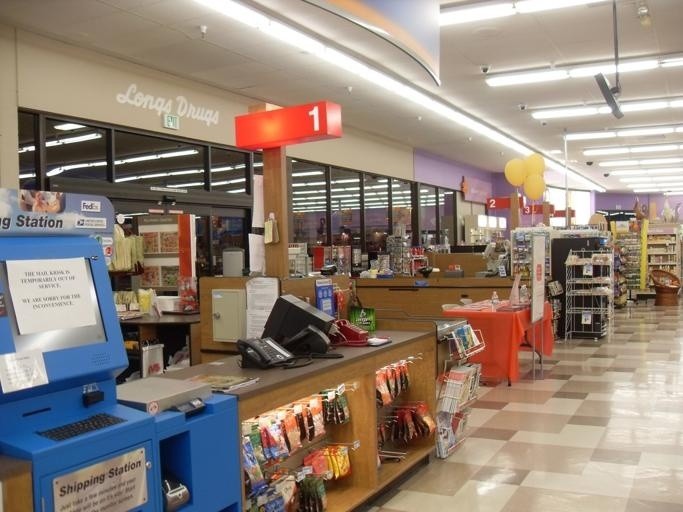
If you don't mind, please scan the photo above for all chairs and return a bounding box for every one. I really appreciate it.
[649,269,680,306]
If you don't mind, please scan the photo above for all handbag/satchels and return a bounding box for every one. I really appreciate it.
[348,306,376,331]
[264,220,280,245]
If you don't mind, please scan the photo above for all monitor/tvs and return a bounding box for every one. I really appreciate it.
[594,73,624,120]
[0,256,109,356]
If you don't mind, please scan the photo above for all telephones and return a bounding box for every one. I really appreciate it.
[236,336,296,369]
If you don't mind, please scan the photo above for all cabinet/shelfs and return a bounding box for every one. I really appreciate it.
[117,310,200,378]
[509,228,551,279]
[564,247,615,344]
[600,232,681,295]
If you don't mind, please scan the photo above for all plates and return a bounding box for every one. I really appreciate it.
[156,296,179,311]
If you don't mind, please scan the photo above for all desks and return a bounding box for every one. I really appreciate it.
[440,298,550,388]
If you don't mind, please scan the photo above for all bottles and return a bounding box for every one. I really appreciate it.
[512,262,529,276]
[351,237,362,267]
[520,285,528,303]
[491,291,498,310]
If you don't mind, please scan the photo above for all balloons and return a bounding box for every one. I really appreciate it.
[523,174,546,201]
[524,154,546,178]
[504,157,525,188]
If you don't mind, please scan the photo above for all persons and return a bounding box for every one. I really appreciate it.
[19,189,65,216]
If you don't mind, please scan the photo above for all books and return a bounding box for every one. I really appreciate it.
[436,323,482,459]
[185,373,260,394]
[647,234,675,285]
